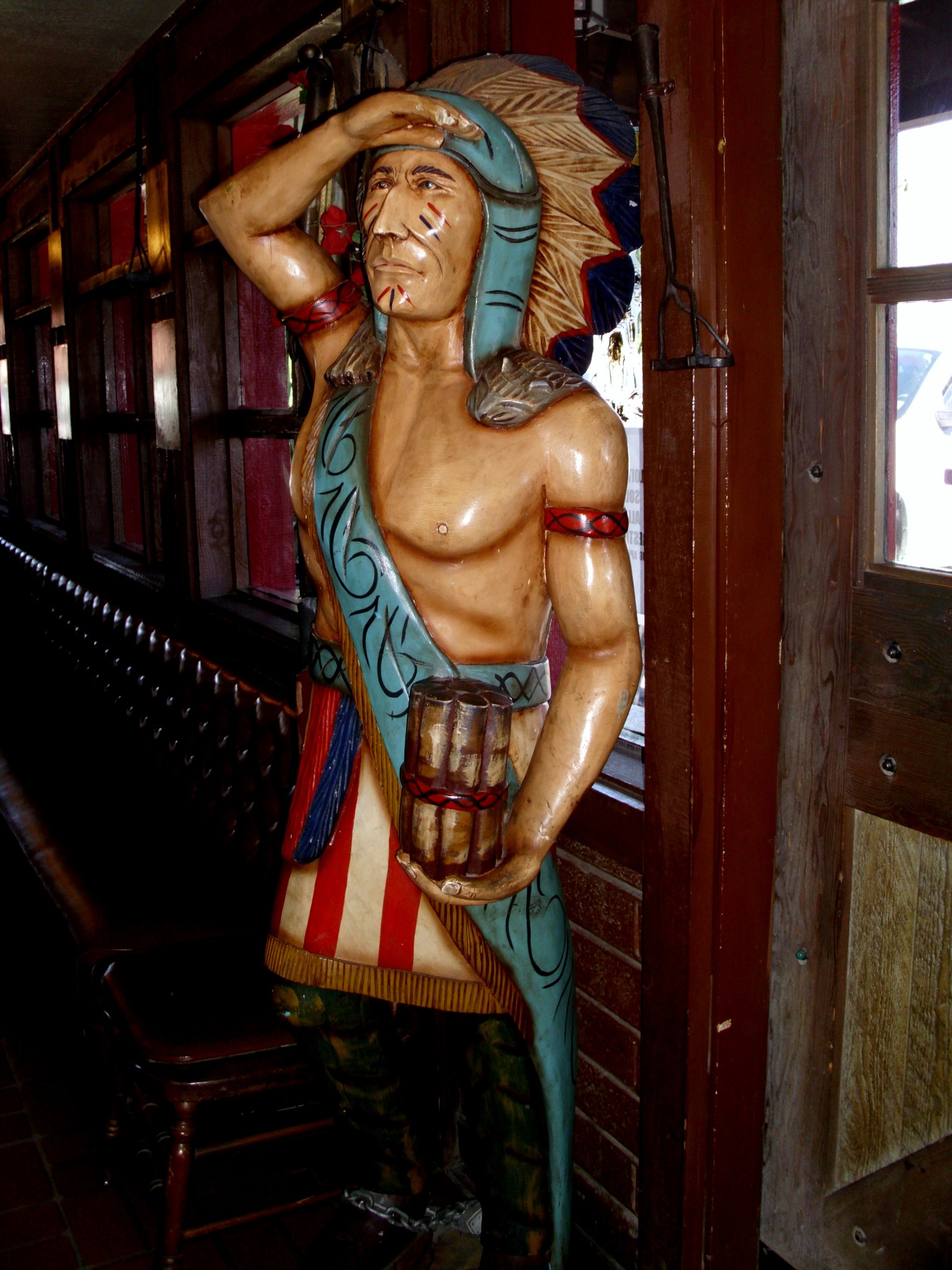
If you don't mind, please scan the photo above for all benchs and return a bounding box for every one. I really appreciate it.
[0,514,355,1270]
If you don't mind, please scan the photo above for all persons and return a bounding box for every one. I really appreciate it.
[191,47,644,1270]
